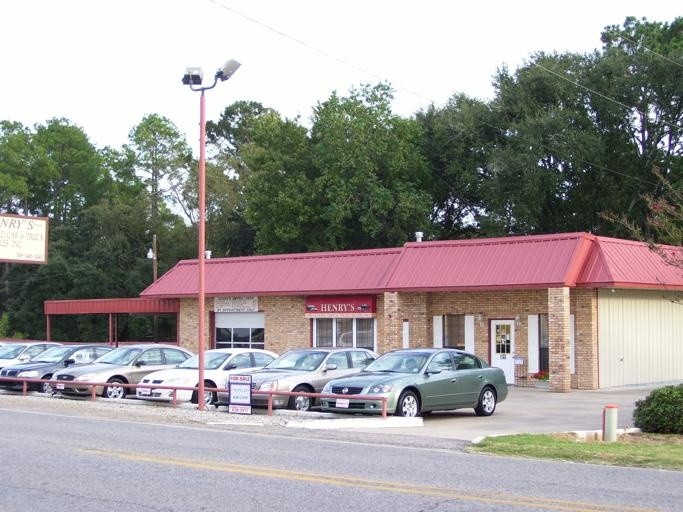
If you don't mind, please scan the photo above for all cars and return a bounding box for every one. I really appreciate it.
[338,329,373,347]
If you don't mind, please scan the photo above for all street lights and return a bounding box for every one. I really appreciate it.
[145,233,158,341]
[181,59,241,411]
